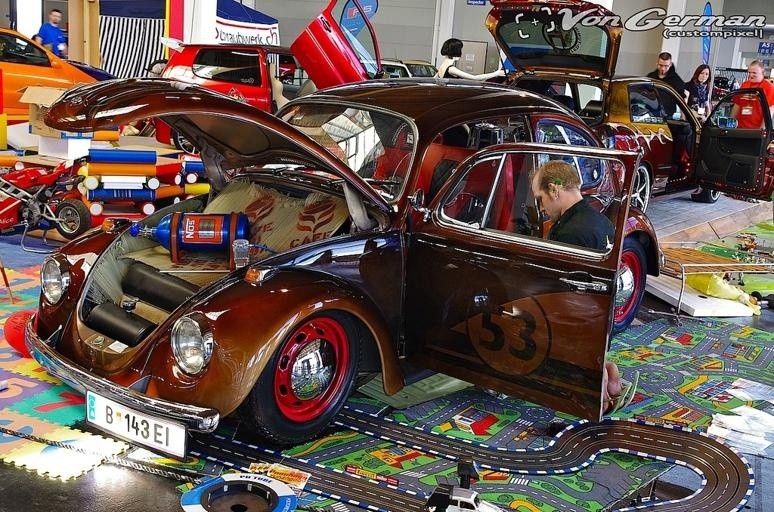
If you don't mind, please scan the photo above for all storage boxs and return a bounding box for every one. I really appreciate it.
[14,85,96,139]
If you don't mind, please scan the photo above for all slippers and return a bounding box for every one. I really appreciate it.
[603,370,639,416]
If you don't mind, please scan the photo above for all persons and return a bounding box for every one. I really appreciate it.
[680,64,710,122]
[730,60,774,129]
[530,161,629,419]
[646,52,686,120]
[25,9,67,58]
[437,38,510,81]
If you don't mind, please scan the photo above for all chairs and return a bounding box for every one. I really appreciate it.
[553,95,604,116]
[269,64,296,110]
[374,127,524,233]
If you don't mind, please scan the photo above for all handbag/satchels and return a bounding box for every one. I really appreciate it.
[685,272,762,316]
